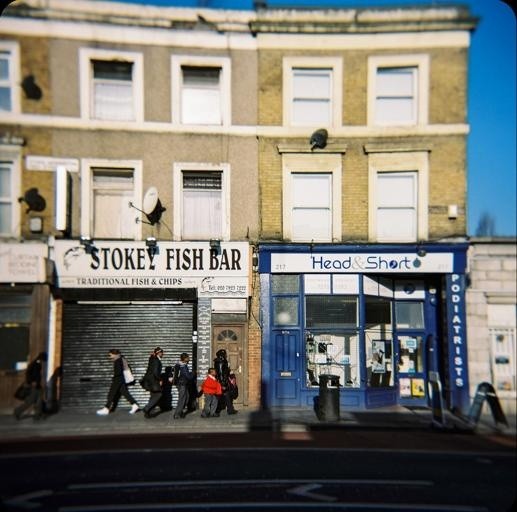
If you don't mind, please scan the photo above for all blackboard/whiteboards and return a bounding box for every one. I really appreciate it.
[468,394,485,425]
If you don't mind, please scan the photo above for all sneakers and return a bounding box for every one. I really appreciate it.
[129,403,139,416]
[95,406,111,416]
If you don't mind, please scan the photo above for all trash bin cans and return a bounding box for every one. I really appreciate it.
[318,374,340,422]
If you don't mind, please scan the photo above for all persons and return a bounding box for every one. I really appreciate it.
[173,353,195,419]
[139,347,165,418]
[14,352,48,421]
[96,349,139,416]
[201,349,239,417]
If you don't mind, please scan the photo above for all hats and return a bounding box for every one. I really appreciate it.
[153,345,163,355]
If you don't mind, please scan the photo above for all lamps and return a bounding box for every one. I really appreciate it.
[208,239,221,254]
[78,236,94,255]
[144,238,157,258]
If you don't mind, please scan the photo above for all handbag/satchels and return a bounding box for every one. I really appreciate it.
[13,385,33,400]
[121,368,134,384]
